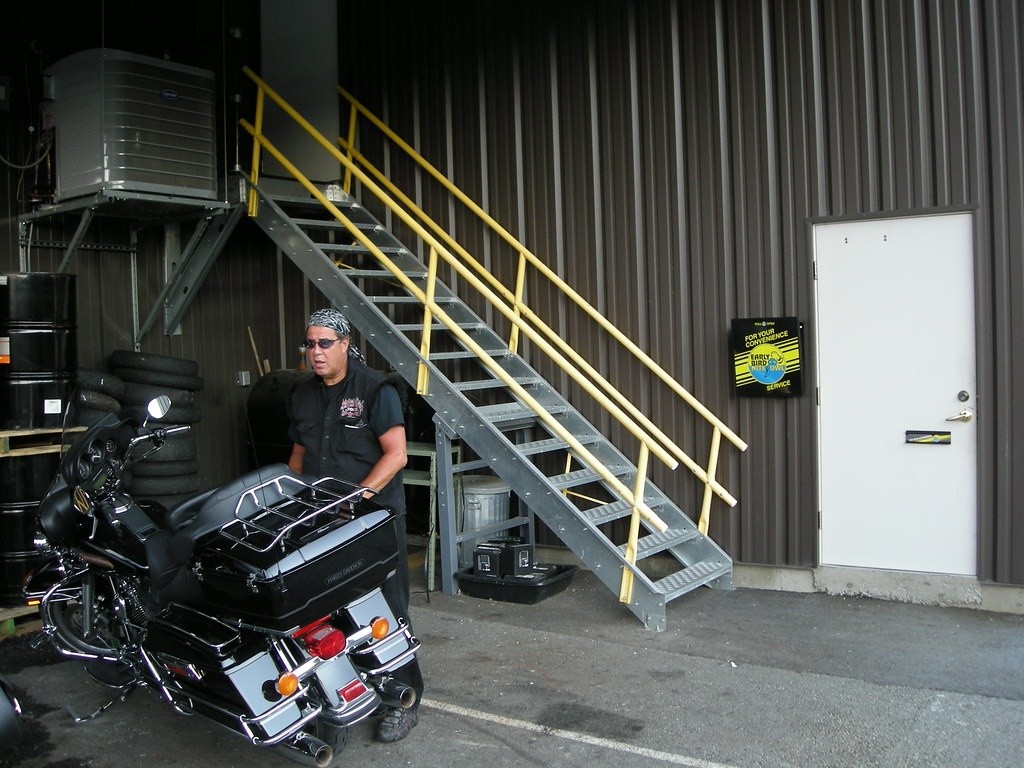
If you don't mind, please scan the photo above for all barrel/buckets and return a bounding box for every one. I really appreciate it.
[453,475,512,569]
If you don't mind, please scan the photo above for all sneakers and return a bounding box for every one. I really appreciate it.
[376,706,419,741]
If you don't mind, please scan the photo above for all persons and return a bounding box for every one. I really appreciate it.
[286,308,424,742]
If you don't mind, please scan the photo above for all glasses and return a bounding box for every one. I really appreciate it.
[301,338,339,349]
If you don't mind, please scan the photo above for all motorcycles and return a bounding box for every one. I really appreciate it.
[20,394,422,768]
